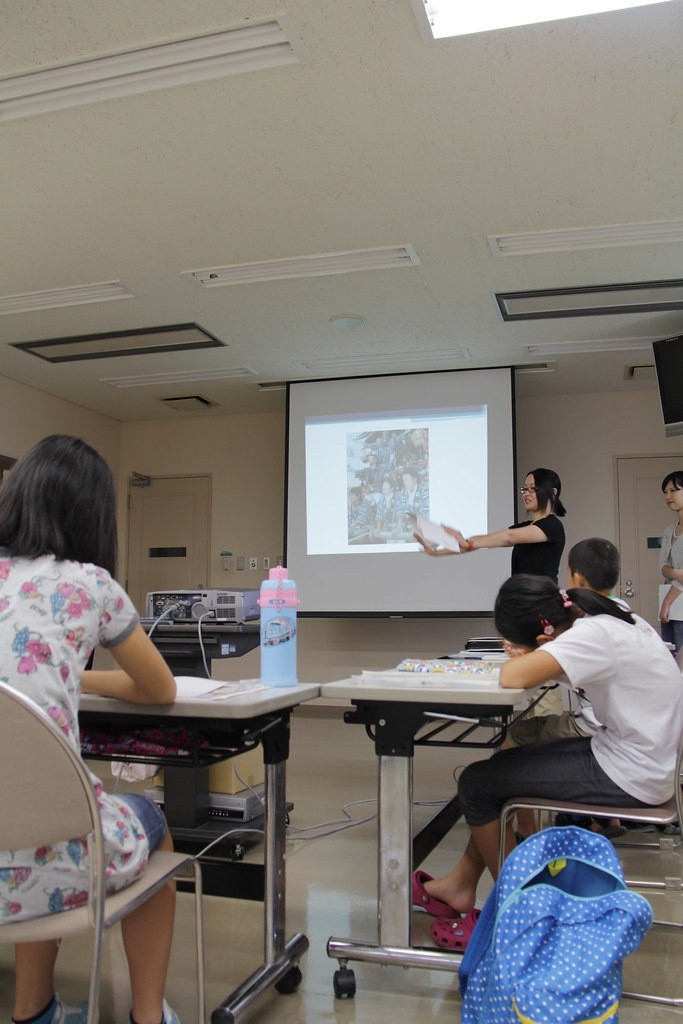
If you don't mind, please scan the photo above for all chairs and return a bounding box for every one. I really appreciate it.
[500,732,683,1006]
[0,679,205,1024]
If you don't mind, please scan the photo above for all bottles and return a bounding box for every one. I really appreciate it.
[259,567,301,687]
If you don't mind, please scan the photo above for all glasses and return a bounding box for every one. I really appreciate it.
[520,485,539,494]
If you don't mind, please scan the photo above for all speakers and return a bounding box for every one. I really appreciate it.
[651,332,683,437]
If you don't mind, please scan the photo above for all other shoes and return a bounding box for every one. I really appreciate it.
[128,997,180,1024]
[12,991,88,1024]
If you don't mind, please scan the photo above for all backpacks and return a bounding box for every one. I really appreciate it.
[458,827,654,1024]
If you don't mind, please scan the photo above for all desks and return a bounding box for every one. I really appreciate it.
[71,679,318,1024]
[319,657,560,1000]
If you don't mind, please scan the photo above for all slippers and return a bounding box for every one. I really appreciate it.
[411,871,460,918]
[430,909,481,950]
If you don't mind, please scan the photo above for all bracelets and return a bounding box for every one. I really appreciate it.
[468,541,473,550]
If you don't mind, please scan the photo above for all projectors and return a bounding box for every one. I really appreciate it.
[145,587,261,623]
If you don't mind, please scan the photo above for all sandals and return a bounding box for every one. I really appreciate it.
[586,817,627,839]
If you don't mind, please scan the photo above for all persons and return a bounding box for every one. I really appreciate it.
[498,537,628,846]
[659,470,683,658]
[349,431,429,545]
[410,574,683,951]
[0,435,180,1024]
[413,468,567,585]
